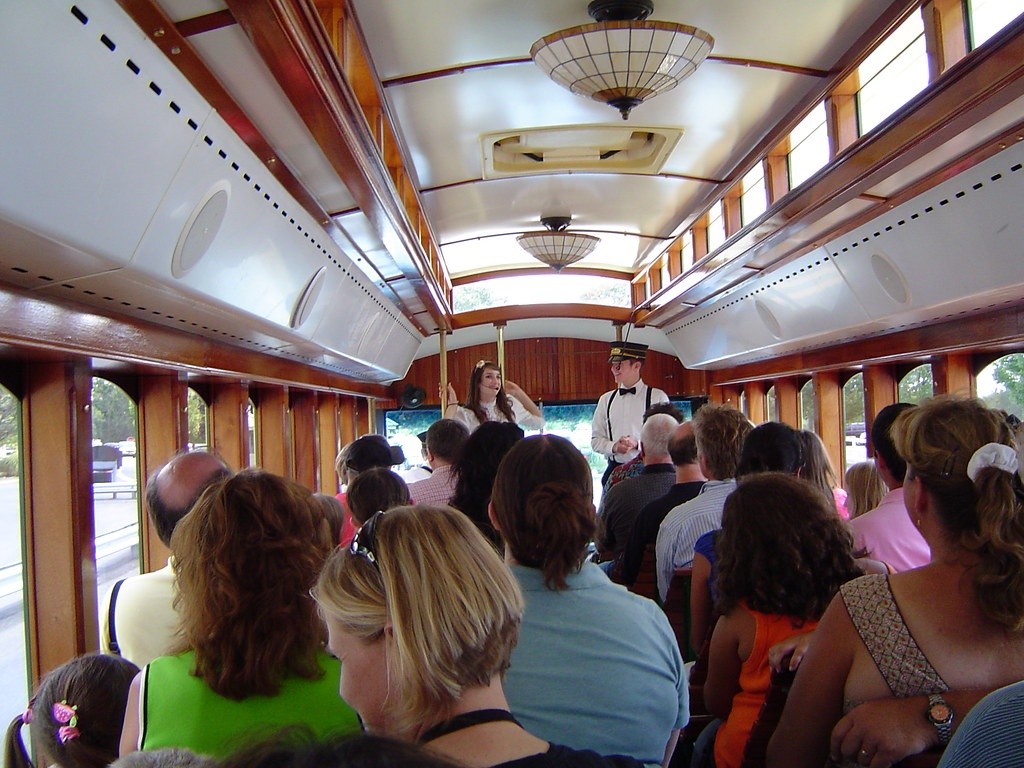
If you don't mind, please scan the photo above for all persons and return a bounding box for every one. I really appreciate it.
[4,390,1024,768]
[590,341,674,487]
[438,360,546,436]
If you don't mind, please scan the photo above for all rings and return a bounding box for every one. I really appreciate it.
[859,746,874,759]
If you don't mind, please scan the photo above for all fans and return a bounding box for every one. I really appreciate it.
[399,383,426,410]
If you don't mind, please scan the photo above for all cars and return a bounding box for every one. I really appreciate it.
[116,440,136,458]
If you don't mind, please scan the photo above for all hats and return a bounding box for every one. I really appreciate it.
[417,431,427,448]
[607,341,649,363]
[344,434,405,470]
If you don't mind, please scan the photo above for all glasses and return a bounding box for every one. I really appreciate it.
[348,511,383,575]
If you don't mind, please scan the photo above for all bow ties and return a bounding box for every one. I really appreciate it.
[619,387,636,396]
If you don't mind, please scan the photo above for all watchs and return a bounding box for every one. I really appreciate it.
[924,691,955,748]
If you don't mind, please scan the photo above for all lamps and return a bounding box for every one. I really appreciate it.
[530,0,715,120]
[516,217,602,273]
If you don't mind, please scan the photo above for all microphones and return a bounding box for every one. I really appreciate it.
[616,306,650,370]
[481,383,499,391]
